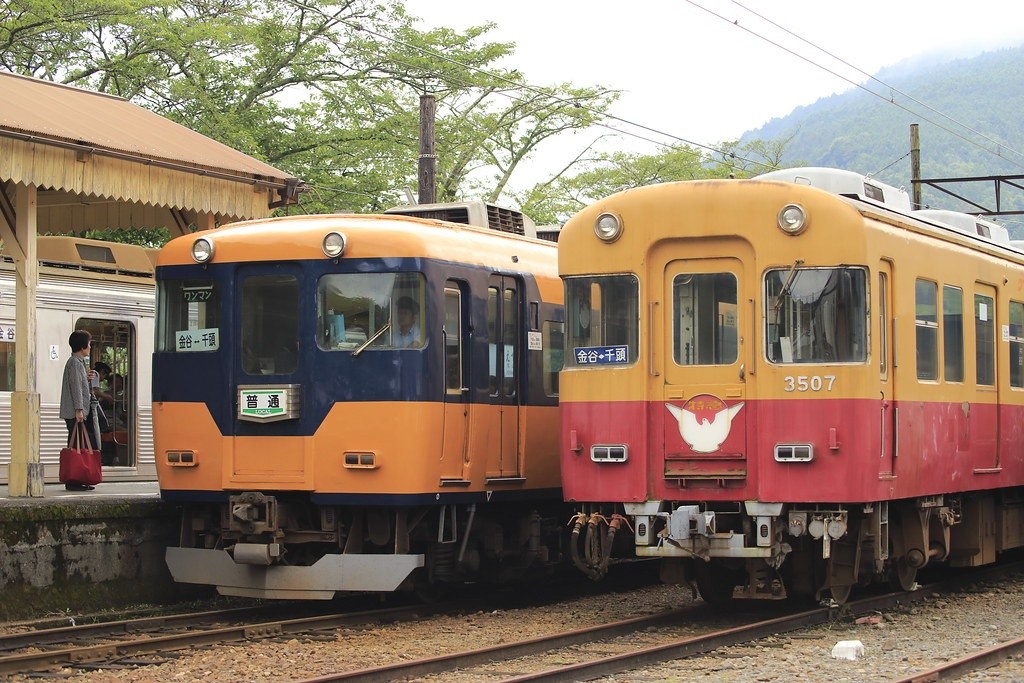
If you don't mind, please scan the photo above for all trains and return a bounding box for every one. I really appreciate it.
[0,236,161,484]
[149,201,776,615]
[556,166,1023,604]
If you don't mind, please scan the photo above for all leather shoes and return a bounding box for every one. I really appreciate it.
[65,483,95,491]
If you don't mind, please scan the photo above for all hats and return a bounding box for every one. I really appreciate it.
[397,296,420,314]
[95,362,112,374]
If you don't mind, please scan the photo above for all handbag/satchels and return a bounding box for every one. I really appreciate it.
[59,419,103,484]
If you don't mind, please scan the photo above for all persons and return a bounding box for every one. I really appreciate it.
[384,295,420,347]
[86,361,129,465]
[58,330,96,490]
